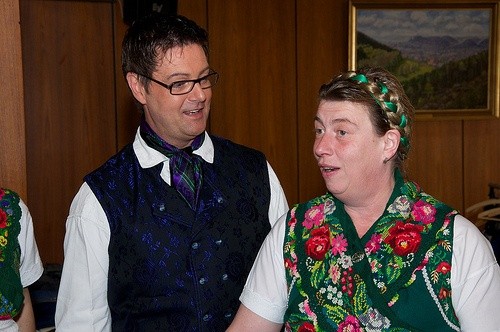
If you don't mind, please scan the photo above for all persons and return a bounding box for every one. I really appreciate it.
[0,188,45,332]
[226,67,500,332]
[54,14,290,332]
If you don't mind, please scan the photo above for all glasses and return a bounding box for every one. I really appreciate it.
[134,68,219,95]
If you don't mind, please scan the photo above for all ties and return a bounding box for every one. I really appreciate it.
[140,121,205,209]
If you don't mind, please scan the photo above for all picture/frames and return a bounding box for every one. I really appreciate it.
[347,0,500,122]
